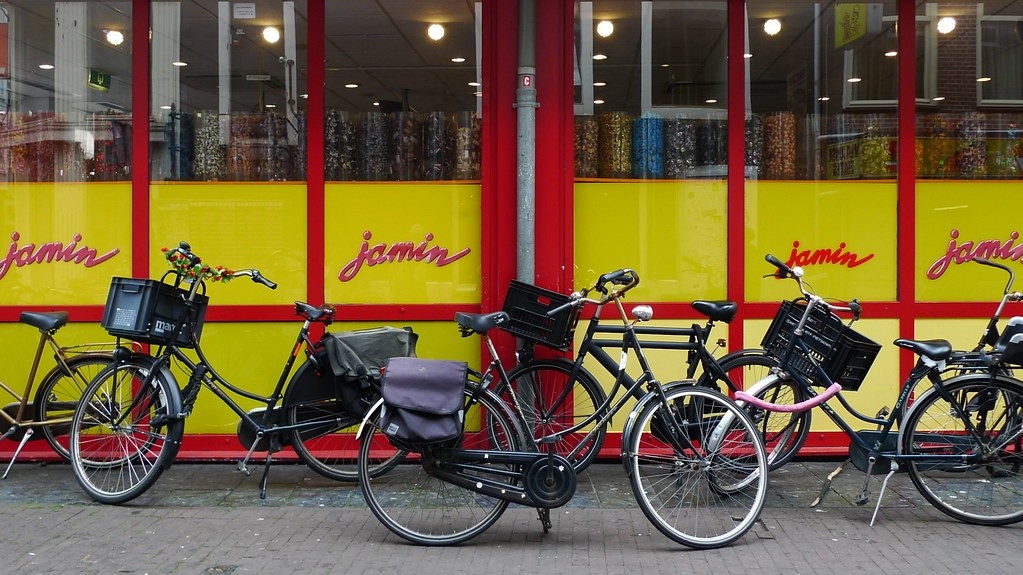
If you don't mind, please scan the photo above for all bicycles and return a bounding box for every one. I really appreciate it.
[705,251,1023,526]
[894,256,1022,474]
[68,242,419,507]
[0,310,160,481]
[488,268,813,480]
[354,268,768,549]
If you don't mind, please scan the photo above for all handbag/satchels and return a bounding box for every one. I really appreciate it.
[380,355,468,445]
[330,329,412,425]
